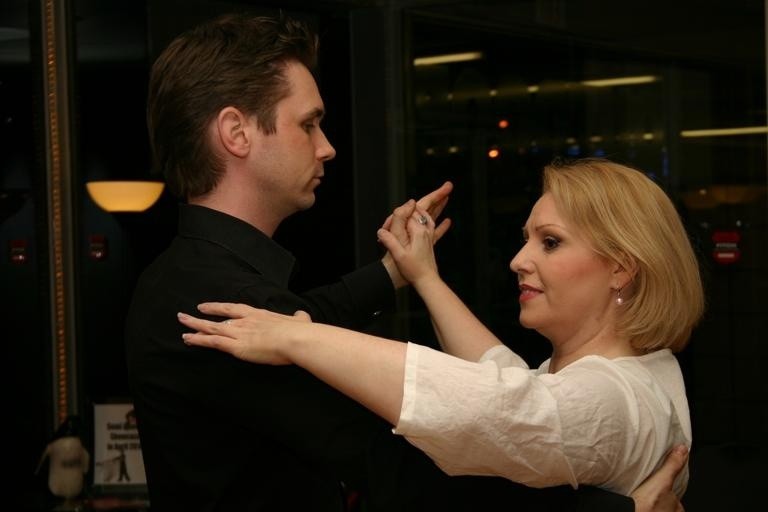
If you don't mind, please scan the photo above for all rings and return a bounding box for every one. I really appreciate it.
[226,319,232,326]
[417,216,428,226]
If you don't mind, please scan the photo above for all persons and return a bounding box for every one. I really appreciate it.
[124,10,690,511]
[176,158,705,501]
